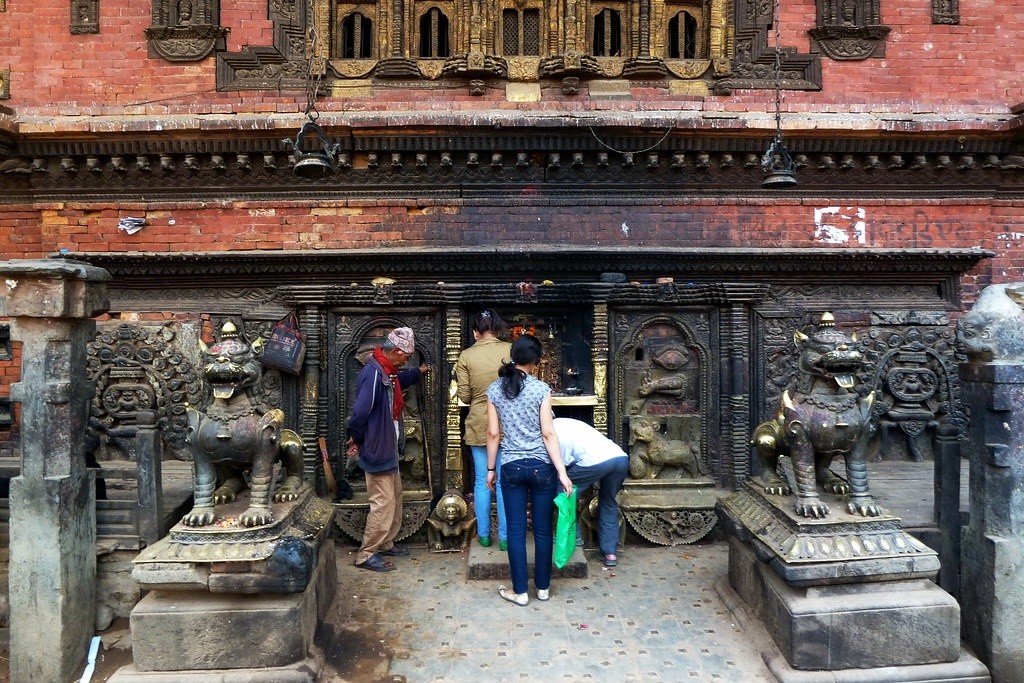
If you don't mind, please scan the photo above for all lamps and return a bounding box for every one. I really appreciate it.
[760,0,796,188]
[280,0,340,179]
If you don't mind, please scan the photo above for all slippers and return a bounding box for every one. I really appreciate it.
[377,546,409,556]
[354,552,396,572]
[602,555,617,566]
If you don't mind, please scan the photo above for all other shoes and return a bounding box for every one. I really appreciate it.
[478,536,492,547]
[553,534,584,546]
[499,585,529,607]
[535,586,549,601]
[499,540,508,551]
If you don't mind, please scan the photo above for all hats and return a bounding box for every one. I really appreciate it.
[388,327,415,354]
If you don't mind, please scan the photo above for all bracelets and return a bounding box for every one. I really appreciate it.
[487,467,495,471]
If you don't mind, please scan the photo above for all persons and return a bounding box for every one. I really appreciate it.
[457,309,514,550]
[551,410,629,565]
[346,328,432,571]
[487,333,574,607]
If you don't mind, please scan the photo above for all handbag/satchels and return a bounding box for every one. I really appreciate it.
[553,484,578,570]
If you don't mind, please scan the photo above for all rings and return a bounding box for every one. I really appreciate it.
[354,449,357,452]
